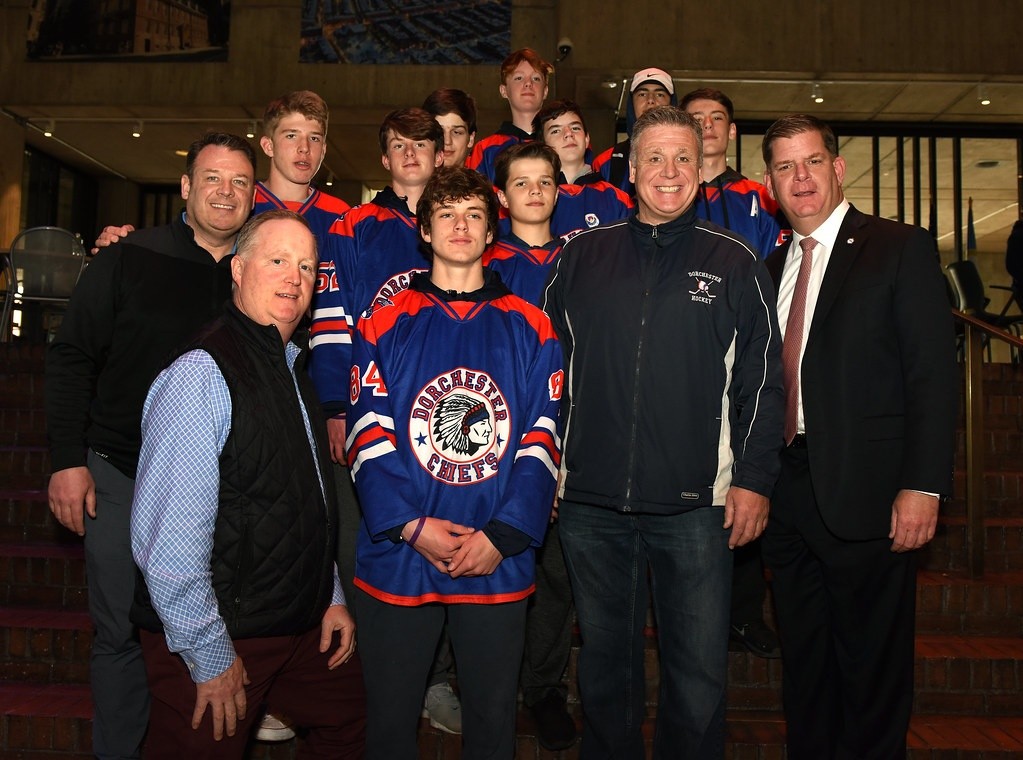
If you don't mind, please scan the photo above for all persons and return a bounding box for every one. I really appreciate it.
[483,137,586,750]
[344,162,569,760]
[307,106,465,735]
[683,88,793,660]
[757,112,963,760]
[530,95,784,760]
[90,90,348,742]
[127,208,357,760]
[422,47,682,241]
[42,132,258,760]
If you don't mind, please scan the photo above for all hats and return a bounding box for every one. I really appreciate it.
[781,237,820,446]
[630,67,675,94]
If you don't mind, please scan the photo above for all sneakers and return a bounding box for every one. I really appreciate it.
[731,616,780,658]
[422,681,463,736]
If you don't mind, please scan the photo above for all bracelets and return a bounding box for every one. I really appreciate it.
[332,415,347,419]
[407,516,426,547]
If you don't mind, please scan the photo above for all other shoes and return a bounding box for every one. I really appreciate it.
[256,713,296,740]
[523,688,578,752]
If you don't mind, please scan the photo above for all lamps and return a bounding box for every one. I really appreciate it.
[44,120,56,137]
[133,121,143,137]
[247,121,257,138]
[327,173,333,186]
[815,88,823,103]
[980,88,990,105]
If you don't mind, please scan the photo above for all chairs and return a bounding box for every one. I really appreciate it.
[0,226,91,342]
[945,260,1022,364]
